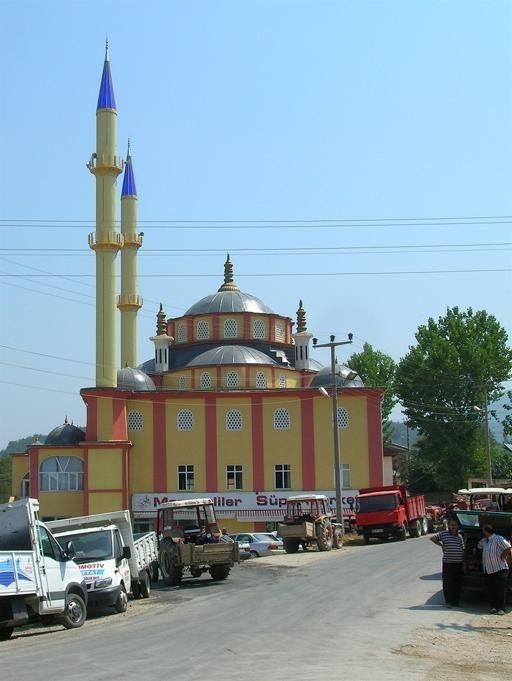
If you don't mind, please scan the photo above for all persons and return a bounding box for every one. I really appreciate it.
[477,524,512,615]
[430,517,467,609]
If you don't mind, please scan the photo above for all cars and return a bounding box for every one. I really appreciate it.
[222,526,303,560]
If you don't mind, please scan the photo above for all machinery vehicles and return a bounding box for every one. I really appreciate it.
[427,497,500,533]
[148,495,242,585]
[276,492,345,552]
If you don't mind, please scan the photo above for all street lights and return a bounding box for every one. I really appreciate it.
[472,404,493,487]
[312,333,361,524]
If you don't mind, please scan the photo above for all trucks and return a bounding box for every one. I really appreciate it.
[0,495,92,644]
[40,508,162,613]
[354,484,431,543]
[443,483,512,599]
